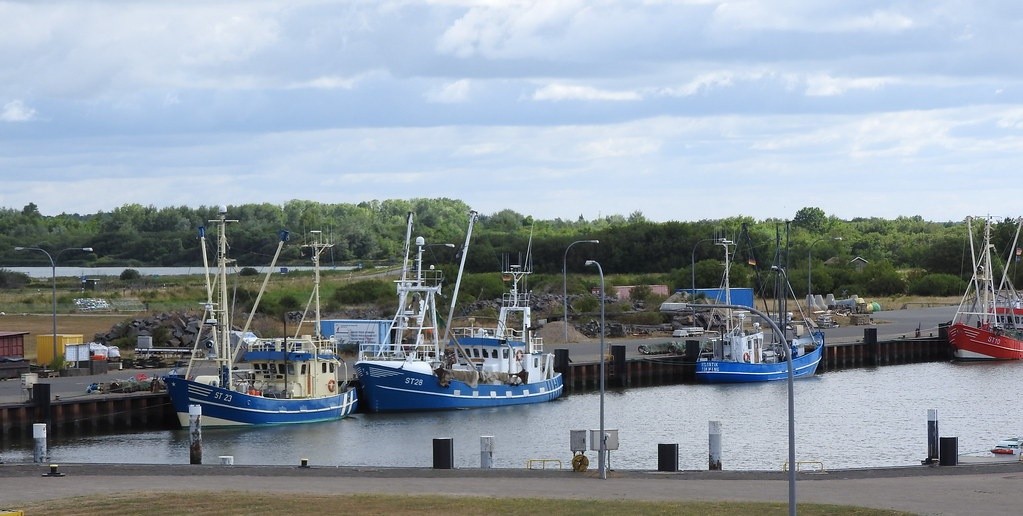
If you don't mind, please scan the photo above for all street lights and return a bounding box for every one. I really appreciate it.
[585,260,607,480]
[14,248,93,372]
[809,237,842,316]
[716,243,729,334]
[693,238,726,326]
[564,240,599,343]
[659,303,796,516]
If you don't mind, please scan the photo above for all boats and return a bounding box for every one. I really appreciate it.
[163,207,358,430]
[695,220,825,383]
[354,210,564,412]
[991,437,1023,458]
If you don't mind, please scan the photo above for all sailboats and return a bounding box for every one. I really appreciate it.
[945,211,1023,360]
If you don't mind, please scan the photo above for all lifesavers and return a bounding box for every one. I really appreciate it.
[744,353,749,361]
[516,350,524,361]
[328,380,335,392]
